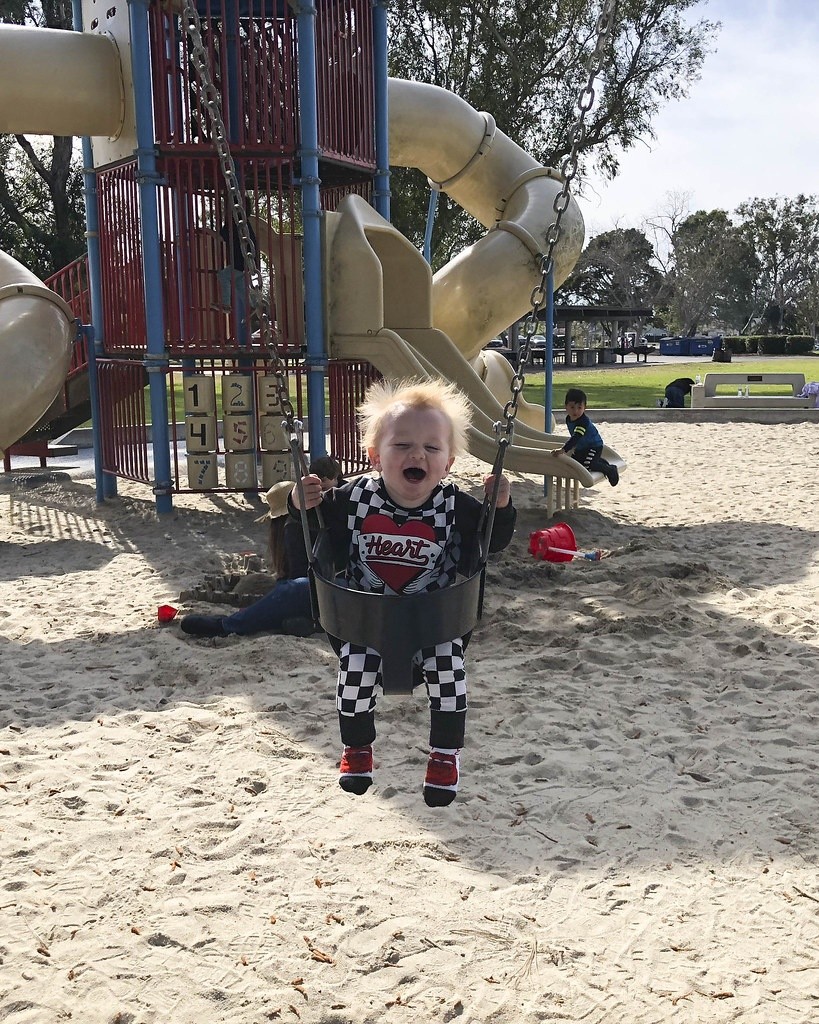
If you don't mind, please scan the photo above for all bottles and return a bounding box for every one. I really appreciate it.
[745,385,749,397]
[696,372,700,385]
[738,384,743,396]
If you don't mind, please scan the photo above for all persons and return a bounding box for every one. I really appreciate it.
[203,193,256,314]
[310,457,349,492]
[180,481,323,637]
[656,377,695,408]
[284,373,519,809]
[797,382,819,408]
[549,388,619,487]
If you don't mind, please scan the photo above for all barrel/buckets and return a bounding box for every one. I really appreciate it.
[529,522,586,563]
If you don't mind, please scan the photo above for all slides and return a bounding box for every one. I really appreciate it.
[1,23,128,456]
[386,75,587,433]
[337,325,631,488]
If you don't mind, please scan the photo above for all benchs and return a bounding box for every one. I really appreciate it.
[704,373,806,398]
[690,384,816,409]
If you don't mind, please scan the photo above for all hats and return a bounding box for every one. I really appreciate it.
[266,480,296,519]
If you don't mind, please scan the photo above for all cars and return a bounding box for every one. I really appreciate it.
[505,335,527,345]
[617,331,649,348]
[483,337,504,348]
[530,335,546,348]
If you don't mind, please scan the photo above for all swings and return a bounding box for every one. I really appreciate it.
[180,0,619,682]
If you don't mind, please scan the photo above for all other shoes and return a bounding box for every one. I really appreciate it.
[661,398,669,408]
[282,616,324,637]
[181,614,229,637]
[605,465,619,487]
[599,458,610,466]
[657,398,662,407]
